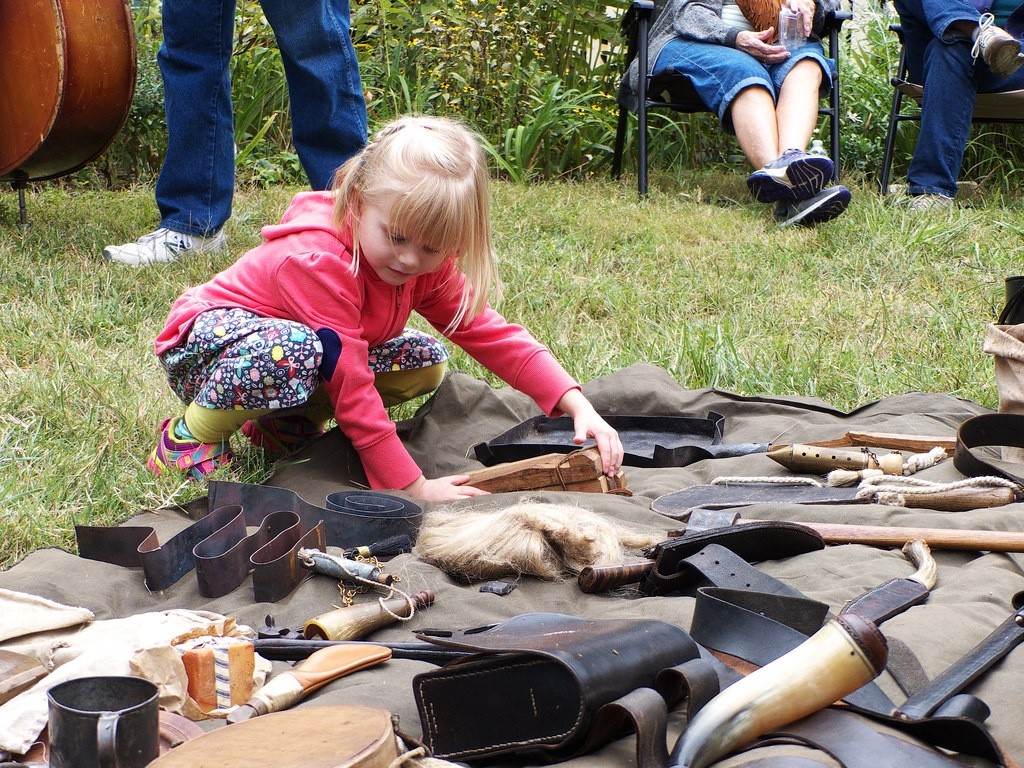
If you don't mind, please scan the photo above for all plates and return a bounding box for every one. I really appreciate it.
[160,709,204,757]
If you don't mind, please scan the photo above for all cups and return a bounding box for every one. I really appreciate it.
[47,676,160,768]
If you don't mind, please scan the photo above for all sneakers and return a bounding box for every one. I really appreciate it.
[104,227,224,267]
[970,12,1024,78]
[907,194,953,214]
[240,404,326,457]
[746,149,833,203]
[149,416,240,484]
[780,185,851,229]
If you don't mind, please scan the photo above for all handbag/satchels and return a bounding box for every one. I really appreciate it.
[415,612,721,768]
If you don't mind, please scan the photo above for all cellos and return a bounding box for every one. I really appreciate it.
[1,0,140,230]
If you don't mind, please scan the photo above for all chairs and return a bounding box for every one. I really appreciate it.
[878,23,1024,201]
[611,0,852,204]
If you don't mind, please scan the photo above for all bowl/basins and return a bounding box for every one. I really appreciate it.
[779,6,808,52]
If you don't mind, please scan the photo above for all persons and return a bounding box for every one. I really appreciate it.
[893,0,1024,212]
[145,115,624,503]
[102,0,370,262]
[628,0,852,232]
